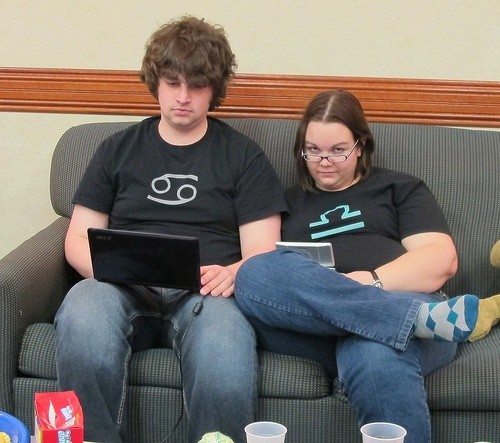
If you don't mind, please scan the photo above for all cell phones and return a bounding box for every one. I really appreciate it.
[275,242,336,272]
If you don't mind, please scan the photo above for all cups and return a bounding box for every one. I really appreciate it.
[244,421,288,443]
[360,422,408,443]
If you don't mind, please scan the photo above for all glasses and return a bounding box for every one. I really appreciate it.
[301,137,361,163]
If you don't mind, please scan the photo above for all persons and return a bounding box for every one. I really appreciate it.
[235,92,479,443]
[52,18,289,443]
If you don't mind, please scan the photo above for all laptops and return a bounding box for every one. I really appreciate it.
[87,228,201,293]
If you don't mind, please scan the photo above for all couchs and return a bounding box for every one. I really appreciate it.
[0,118,500,443]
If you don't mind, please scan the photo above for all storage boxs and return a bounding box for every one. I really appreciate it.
[32,390,85,443]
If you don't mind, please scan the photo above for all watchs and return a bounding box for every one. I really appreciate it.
[370,270,382,289]
